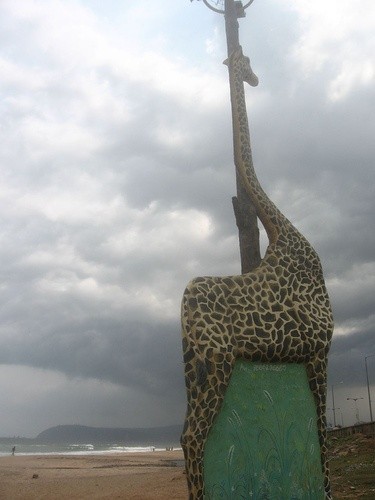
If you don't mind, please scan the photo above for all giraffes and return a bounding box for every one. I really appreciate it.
[180,45,334,495]
